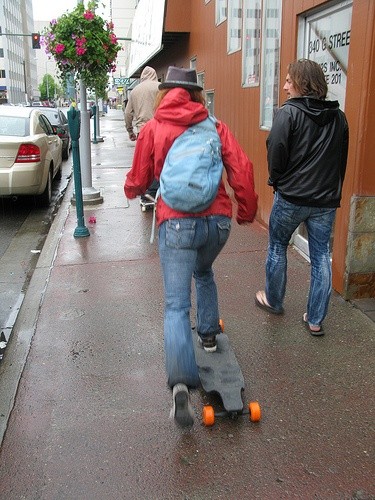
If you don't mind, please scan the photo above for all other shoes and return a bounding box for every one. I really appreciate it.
[169,384,197,428]
[139,192,156,205]
[195,331,219,353]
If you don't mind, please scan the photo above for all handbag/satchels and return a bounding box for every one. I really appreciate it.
[161,114,224,211]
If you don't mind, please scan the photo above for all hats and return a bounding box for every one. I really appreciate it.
[157,66,204,92]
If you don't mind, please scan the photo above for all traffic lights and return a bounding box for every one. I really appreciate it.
[32,33,41,49]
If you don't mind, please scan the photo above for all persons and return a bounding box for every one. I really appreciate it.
[123,66,258,429]
[71,100,76,109]
[254,58,350,336]
[124,66,161,202]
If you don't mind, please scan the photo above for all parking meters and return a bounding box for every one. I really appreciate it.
[66,105,90,237]
[90,105,99,144]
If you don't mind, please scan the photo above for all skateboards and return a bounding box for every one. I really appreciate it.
[185,318,262,426]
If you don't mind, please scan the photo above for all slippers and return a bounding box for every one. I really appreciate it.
[301,311,326,336]
[256,290,285,314]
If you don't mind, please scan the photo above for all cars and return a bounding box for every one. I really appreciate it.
[78,100,95,119]
[0,105,65,208]
[27,106,72,161]
[1,101,70,108]
